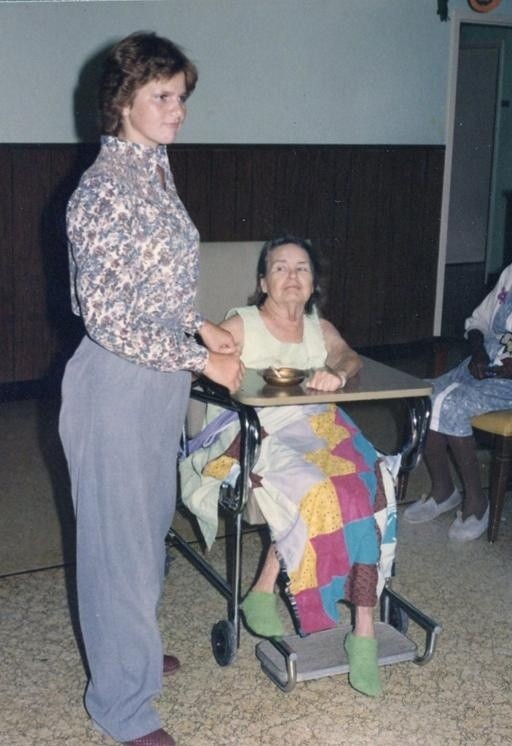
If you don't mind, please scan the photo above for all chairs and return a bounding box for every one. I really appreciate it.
[448,502,490,541]
[404,486,458,523]
[128,728,175,746]
[164,655,180,676]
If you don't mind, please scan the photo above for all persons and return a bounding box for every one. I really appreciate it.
[206,234,385,701]
[400,263,511,544]
[51,28,249,746]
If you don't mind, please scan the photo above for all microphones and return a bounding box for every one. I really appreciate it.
[398,409,512,543]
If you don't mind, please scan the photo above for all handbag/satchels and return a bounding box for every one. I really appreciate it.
[325,362,347,388]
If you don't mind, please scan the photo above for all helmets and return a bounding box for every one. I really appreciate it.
[259,367,307,388]
[261,382,302,398]
